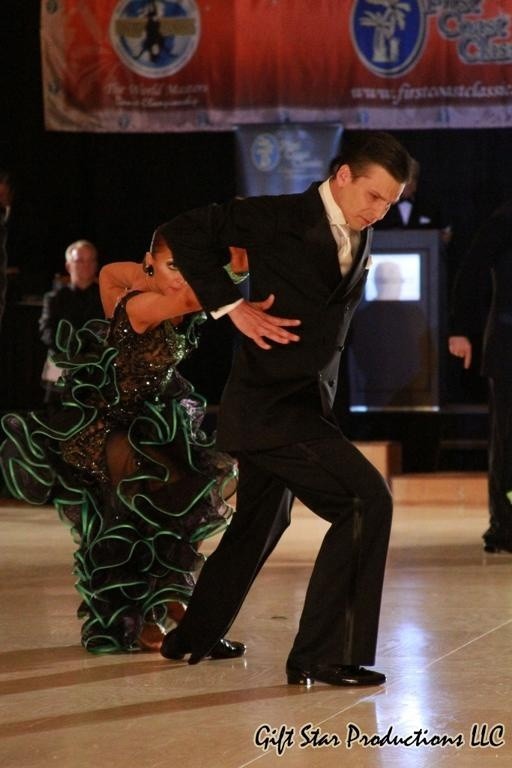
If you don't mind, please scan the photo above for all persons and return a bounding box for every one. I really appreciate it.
[0,175,41,421]
[38,239,106,505]
[370,151,454,229]
[59,222,251,654]
[160,129,422,685]
[443,198,512,554]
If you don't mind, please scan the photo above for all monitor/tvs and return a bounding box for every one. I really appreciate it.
[364,251,424,304]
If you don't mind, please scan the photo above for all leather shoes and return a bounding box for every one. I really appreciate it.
[159,632,246,660]
[285,652,387,688]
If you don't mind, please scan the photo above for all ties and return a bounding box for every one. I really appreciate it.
[336,221,358,275]
